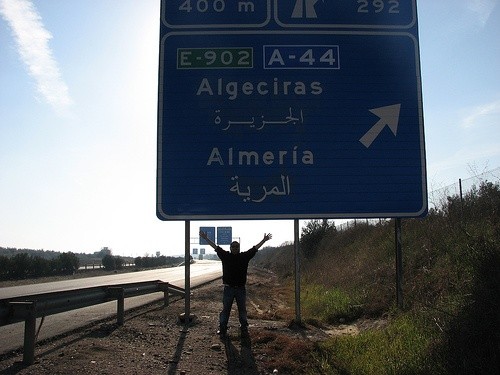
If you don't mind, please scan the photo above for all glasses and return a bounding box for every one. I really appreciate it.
[230,245,239,248]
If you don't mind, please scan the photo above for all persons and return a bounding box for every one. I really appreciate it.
[199,230,273,336]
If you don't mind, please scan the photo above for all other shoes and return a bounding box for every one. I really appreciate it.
[216,326,227,334]
[239,317,249,327]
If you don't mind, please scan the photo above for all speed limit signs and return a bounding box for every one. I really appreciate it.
[155,0,430,221]
